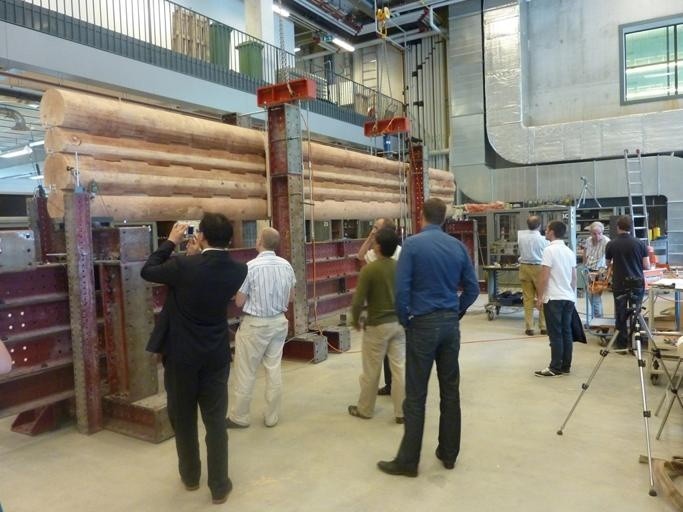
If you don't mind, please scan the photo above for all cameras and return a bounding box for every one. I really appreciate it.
[187,225,195,238]
[622,275,643,291]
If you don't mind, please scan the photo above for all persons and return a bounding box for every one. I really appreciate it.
[518,215,549,335]
[225,227,297,429]
[140,212,248,504]
[347,227,407,424]
[358,217,404,396]
[583,222,614,318]
[605,215,651,354]
[377,198,480,478]
[534,220,578,377]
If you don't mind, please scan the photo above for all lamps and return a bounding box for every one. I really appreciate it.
[330,35,355,52]
[0,120,44,179]
[272,4,290,18]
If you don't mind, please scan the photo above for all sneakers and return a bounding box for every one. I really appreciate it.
[525,329,534,336]
[534,367,570,378]
[540,330,548,334]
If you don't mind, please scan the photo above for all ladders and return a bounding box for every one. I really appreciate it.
[619,148,652,247]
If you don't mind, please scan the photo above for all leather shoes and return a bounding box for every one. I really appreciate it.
[377,459,418,477]
[263,415,279,427]
[395,416,405,424]
[435,448,454,469]
[226,417,250,429]
[181,476,199,490]
[212,478,233,504]
[377,384,392,395]
[348,405,371,419]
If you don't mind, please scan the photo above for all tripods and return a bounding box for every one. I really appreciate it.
[555,291,683,498]
[577,181,602,209]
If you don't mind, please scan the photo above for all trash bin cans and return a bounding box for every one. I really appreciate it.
[235,39,265,81]
[211,21,234,76]
